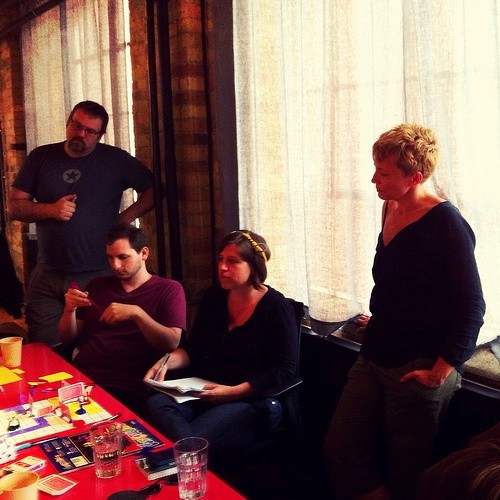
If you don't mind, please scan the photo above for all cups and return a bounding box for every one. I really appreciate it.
[173,437,208,500]
[0,470,40,500]
[89,421,122,478]
[0,337,23,367]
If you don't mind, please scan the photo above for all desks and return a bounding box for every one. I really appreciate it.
[0,304,248,500]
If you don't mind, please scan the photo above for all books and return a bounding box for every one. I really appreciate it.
[40,418,163,478]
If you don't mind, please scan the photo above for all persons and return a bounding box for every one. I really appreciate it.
[143,229,300,475]
[7,101,166,350]
[58,226,188,412]
[314,123,486,500]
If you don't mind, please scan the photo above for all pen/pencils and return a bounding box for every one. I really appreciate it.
[71,196,76,204]
[152,352,170,381]
[71,281,103,313]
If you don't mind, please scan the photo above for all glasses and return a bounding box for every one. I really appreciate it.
[71,118,101,136]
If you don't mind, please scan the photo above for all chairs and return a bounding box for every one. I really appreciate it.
[170,282,304,500]
